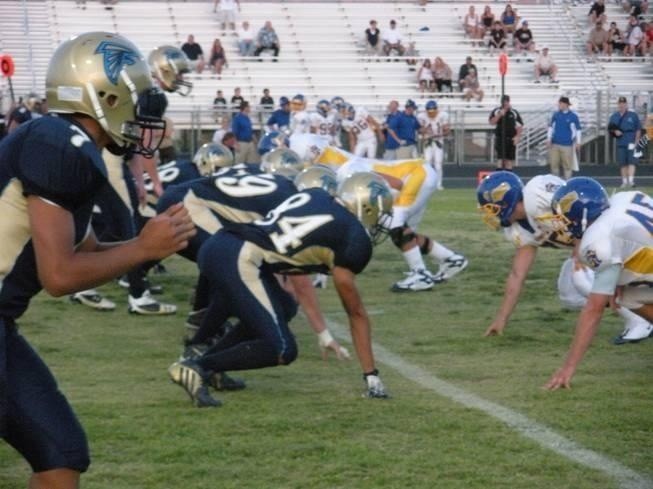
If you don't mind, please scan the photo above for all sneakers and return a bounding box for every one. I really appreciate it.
[69,288,116,312]
[613,320,652,344]
[127,289,177,316]
[166,361,222,408]
[620,182,628,189]
[430,252,468,284]
[179,344,246,392]
[629,182,635,189]
[117,276,162,294]
[390,268,434,293]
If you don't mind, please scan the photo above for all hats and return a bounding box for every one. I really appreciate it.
[558,97,571,105]
[279,97,290,106]
[618,96,626,103]
[404,99,417,110]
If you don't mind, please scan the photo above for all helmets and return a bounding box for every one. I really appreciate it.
[43,31,170,158]
[331,97,343,106]
[334,171,394,247]
[474,170,524,232]
[147,44,192,97]
[191,140,235,177]
[339,102,355,120]
[316,99,330,118]
[262,147,305,182]
[533,175,610,240]
[426,101,437,110]
[294,163,340,197]
[290,95,306,112]
[256,131,290,156]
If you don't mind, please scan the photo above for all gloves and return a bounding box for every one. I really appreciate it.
[318,329,350,360]
[313,272,328,289]
[365,371,387,397]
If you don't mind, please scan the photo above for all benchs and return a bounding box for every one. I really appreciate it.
[0,0,652,131]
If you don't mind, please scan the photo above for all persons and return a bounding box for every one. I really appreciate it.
[213,1,242,37]
[500,4,517,36]
[513,20,533,54]
[533,45,558,84]
[488,96,524,170]
[206,39,227,80]
[402,39,421,73]
[388,99,431,160]
[417,101,450,191]
[230,101,259,165]
[476,173,653,345]
[263,96,289,135]
[212,89,229,125]
[305,144,468,292]
[540,176,653,389]
[339,103,384,159]
[546,96,582,180]
[384,21,405,63]
[166,172,394,407]
[199,150,303,180]
[154,166,348,363]
[433,56,454,100]
[1,104,32,141]
[236,23,256,63]
[464,4,481,41]
[289,96,325,137]
[481,5,494,36]
[461,66,484,111]
[221,130,237,165]
[332,96,351,130]
[418,58,435,100]
[230,87,243,118]
[213,117,230,142]
[362,19,383,63]
[381,101,402,162]
[607,97,649,189]
[458,56,479,92]
[1,31,198,485]
[153,113,177,166]
[583,0,653,62]
[258,89,277,118]
[182,33,204,74]
[488,22,506,55]
[310,99,334,147]
[255,19,281,64]
[68,46,193,314]
[118,142,231,291]
